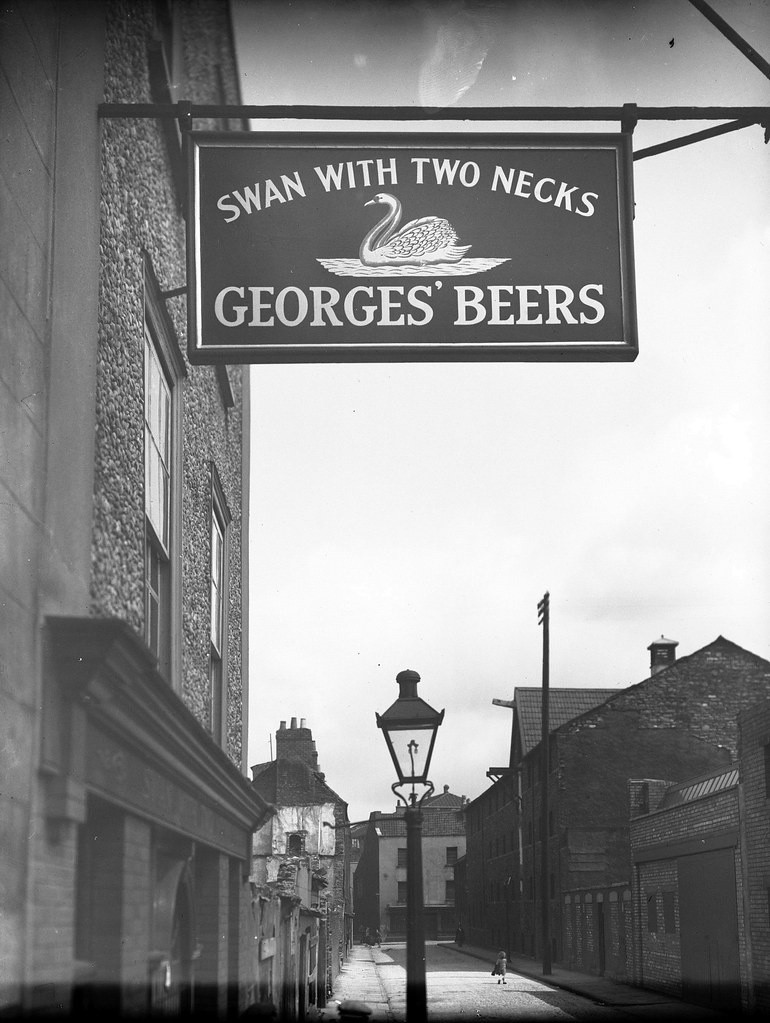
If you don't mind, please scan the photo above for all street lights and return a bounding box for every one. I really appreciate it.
[375,669,446,1022]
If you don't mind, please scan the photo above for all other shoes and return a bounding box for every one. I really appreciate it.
[503,981,507,984]
[498,979,500,984]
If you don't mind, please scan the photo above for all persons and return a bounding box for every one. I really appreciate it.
[457,924,464,947]
[375,928,381,948]
[496,952,507,984]
[241,1002,371,1023]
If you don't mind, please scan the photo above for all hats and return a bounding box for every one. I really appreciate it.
[499,951,506,957]
